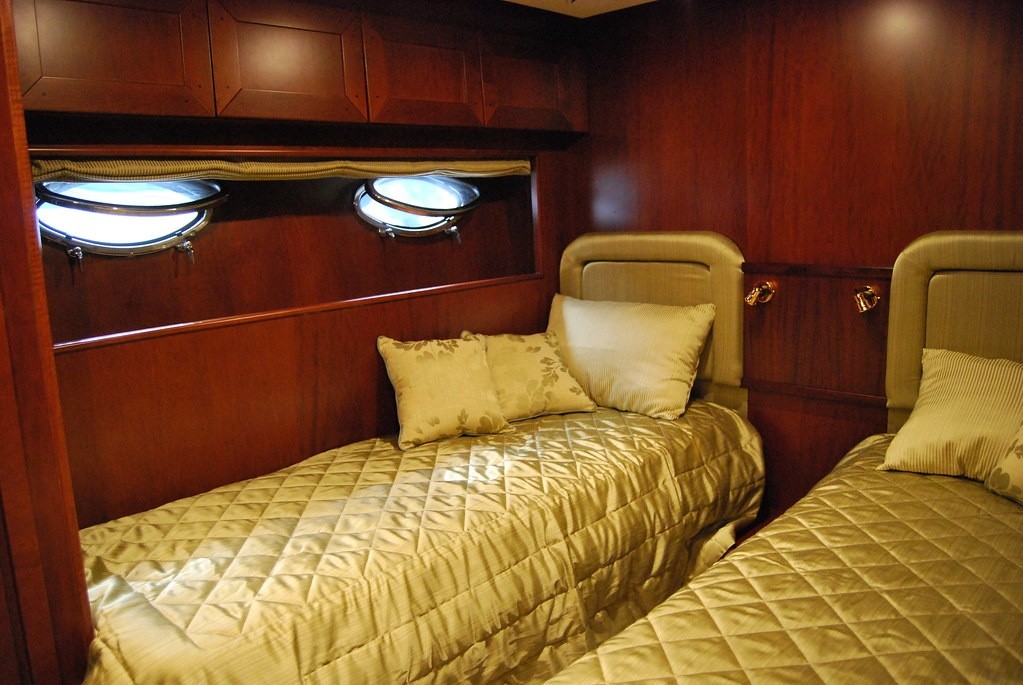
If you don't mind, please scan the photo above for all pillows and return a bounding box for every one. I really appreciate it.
[985,421,1023,505]
[548,293,716,420]
[375,334,516,450]
[876,348,1023,482]
[462,328,596,425]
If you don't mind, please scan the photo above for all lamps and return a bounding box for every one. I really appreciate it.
[744,279,775,306]
[854,287,878,314]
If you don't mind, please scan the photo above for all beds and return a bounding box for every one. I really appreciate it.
[545,228,1023,685]
[80,229,765,685]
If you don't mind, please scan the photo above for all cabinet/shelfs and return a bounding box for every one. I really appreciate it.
[11,0,591,149]
